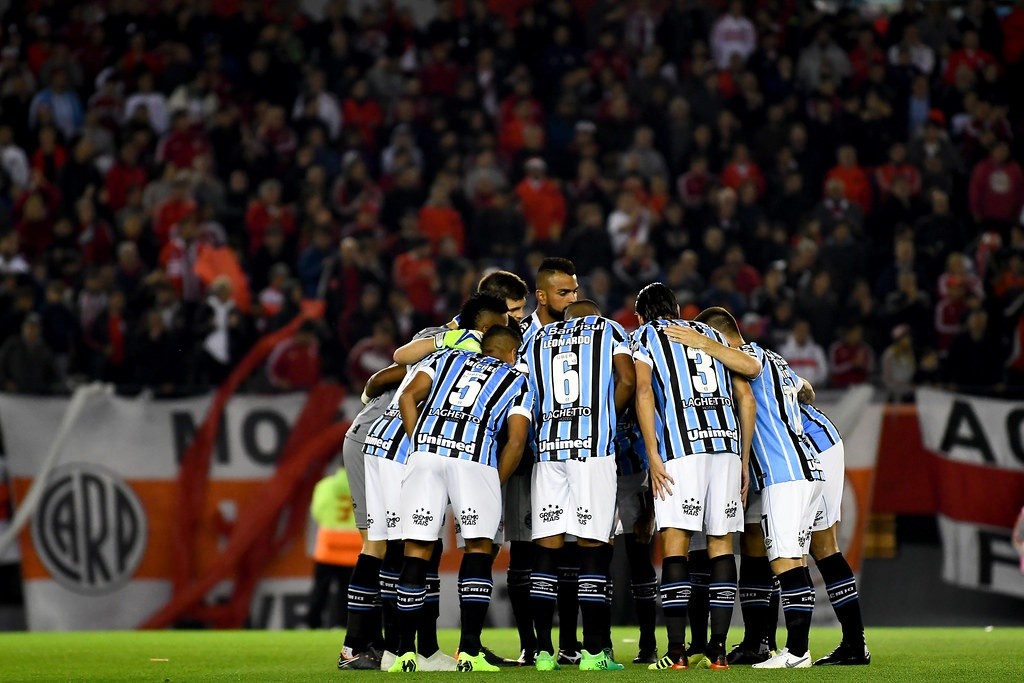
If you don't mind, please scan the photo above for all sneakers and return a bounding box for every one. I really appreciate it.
[558,649,581,665]
[579,648,624,671]
[604,649,615,661]
[648,652,690,670]
[727,638,772,665]
[518,641,536,666]
[814,641,872,666]
[336,646,516,672]
[538,651,560,671]
[695,647,728,670]
[734,643,777,651]
[751,647,813,669]
[686,642,708,663]
[633,649,657,663]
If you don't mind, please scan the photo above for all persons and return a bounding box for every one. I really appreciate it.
[337,257,872,672]
[0,0,1024,400]
[300,452,364,629]
